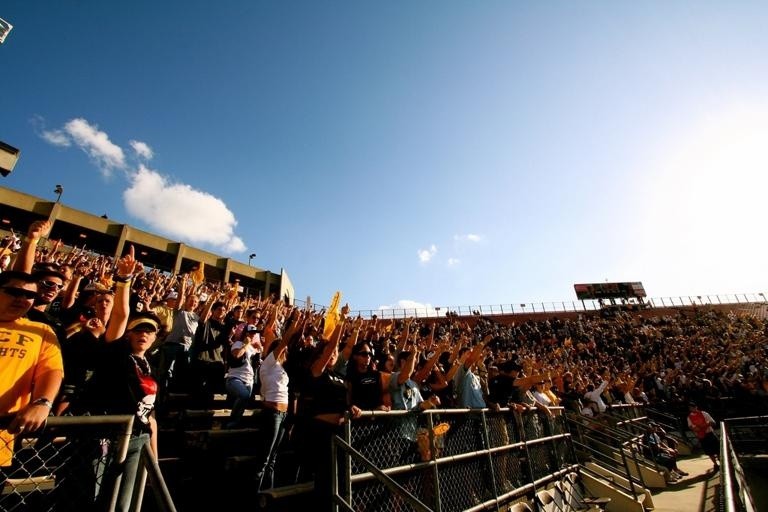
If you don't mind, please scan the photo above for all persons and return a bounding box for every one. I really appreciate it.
[1,219,767,512]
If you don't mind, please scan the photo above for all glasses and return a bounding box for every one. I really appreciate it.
[38,279,64,291]
[535,382,544,385]
[0,285,39,299]
[355,351,372,357]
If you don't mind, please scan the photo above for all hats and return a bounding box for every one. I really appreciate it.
[83,282,112,294]
[242,324,259,333]
[165,292,177,300]
[126,317,159,334]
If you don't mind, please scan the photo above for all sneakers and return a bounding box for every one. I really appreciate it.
[666,469,688,482]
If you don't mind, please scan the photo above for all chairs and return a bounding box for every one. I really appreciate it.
[507,471,612,512]
